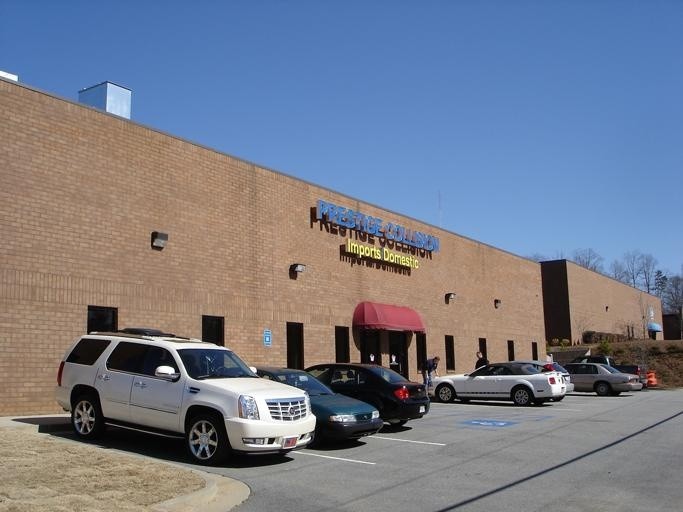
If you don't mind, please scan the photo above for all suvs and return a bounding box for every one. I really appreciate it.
[52,325,316,466]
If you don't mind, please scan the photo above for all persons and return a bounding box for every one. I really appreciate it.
[475,351,489,371]
[421,355,439,388]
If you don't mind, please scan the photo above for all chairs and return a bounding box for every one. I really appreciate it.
[332,371,355,386]
[183,355,198,379]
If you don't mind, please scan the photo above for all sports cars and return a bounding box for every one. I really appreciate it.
[434,363,566,406]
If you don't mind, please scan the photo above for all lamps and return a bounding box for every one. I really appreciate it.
[151,229,169,250]
[446,291,456,300]
[291,262,308,274]
[494,299,502,310]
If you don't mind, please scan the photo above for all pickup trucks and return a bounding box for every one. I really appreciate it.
[571,355,648,389]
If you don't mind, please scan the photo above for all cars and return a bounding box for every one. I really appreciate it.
[490,360,574,402]
[561,362,643,396]
[303,362,430,429]
[251,365,384,449]
